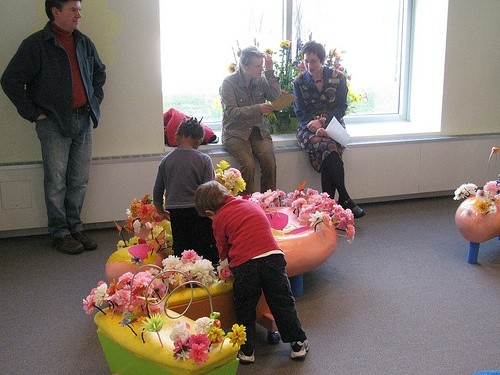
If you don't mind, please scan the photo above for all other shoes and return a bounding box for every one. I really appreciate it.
[343,198,365,218]
[267,331,281,343]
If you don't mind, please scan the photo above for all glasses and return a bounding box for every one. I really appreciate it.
[302,58,320,65]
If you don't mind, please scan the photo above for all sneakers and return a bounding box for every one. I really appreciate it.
[71,231,98,250]
[290,340,310,358]
[236,346,255,362]
[52,234,84,254]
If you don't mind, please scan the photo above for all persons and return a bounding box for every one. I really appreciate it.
[294,41,365,219]
[218,45,283,196]
[0,0,106,255]
[153,117,220,271]
[194,180,309,363]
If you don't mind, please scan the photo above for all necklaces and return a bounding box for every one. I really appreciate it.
[315,79,321,83]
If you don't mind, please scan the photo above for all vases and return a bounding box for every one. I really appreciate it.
[267,115,298,133]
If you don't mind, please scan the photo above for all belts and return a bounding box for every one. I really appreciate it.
[72,105,90,114]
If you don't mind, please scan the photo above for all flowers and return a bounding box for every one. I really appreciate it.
[215,37,366,119]
[82,160,355,365]
[453,173,500,215]
